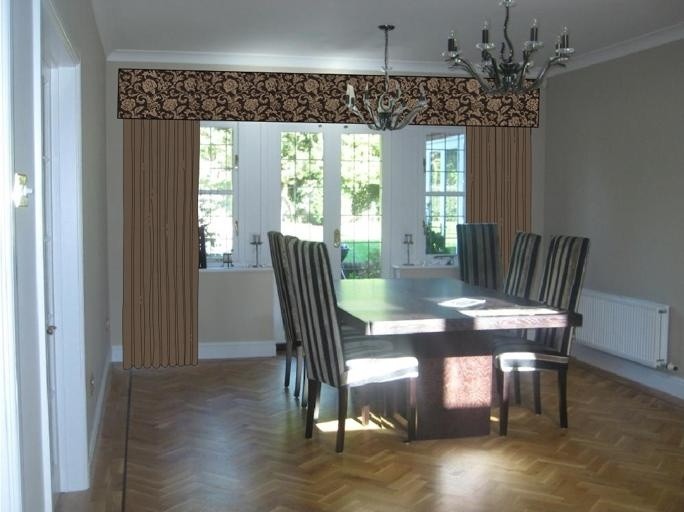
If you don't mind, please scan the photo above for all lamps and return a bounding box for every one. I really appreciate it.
[249,235,264,268]
[440,0,577,97]
[341,24,430,132]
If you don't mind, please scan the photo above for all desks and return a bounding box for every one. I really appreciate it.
[330,277,584,442]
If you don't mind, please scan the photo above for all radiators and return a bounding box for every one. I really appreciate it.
[571,287,680,373]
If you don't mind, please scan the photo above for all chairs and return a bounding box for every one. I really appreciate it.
[495,236,589,435]
[282,236,420,450]
[269,230,395,397]
[500,232,540,404]
[456,225,498,294]
